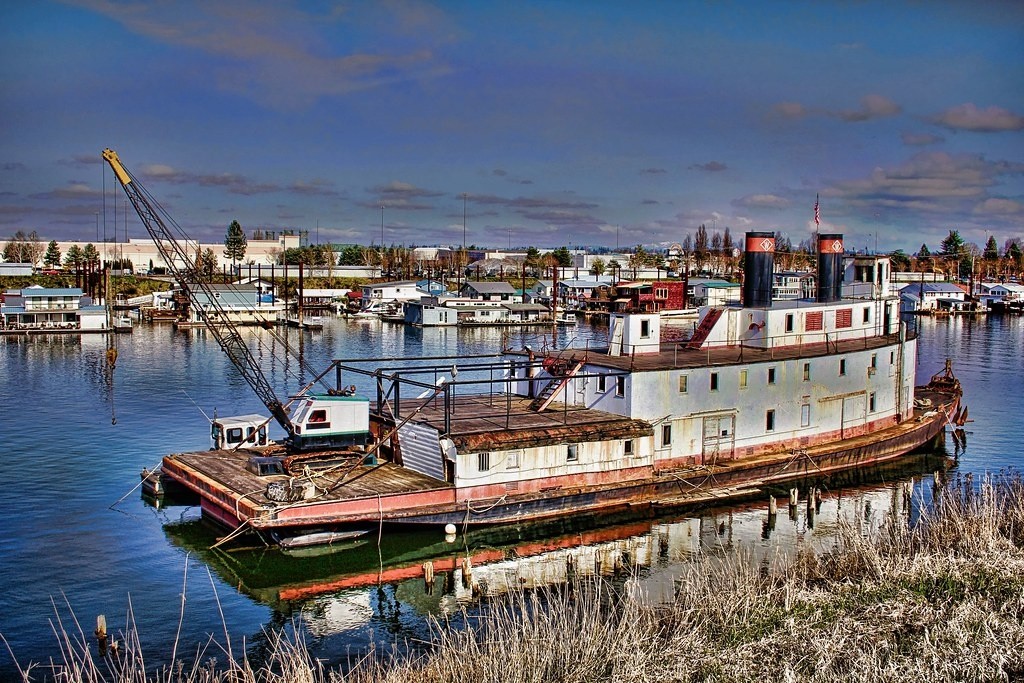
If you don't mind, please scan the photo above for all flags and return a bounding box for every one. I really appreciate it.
[814,197,820,225]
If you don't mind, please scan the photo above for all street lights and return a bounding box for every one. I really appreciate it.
[380,206,387,245]
[460,190,470,248]
[314,218,321,244]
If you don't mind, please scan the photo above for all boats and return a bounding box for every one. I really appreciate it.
[146,228,970,601]
